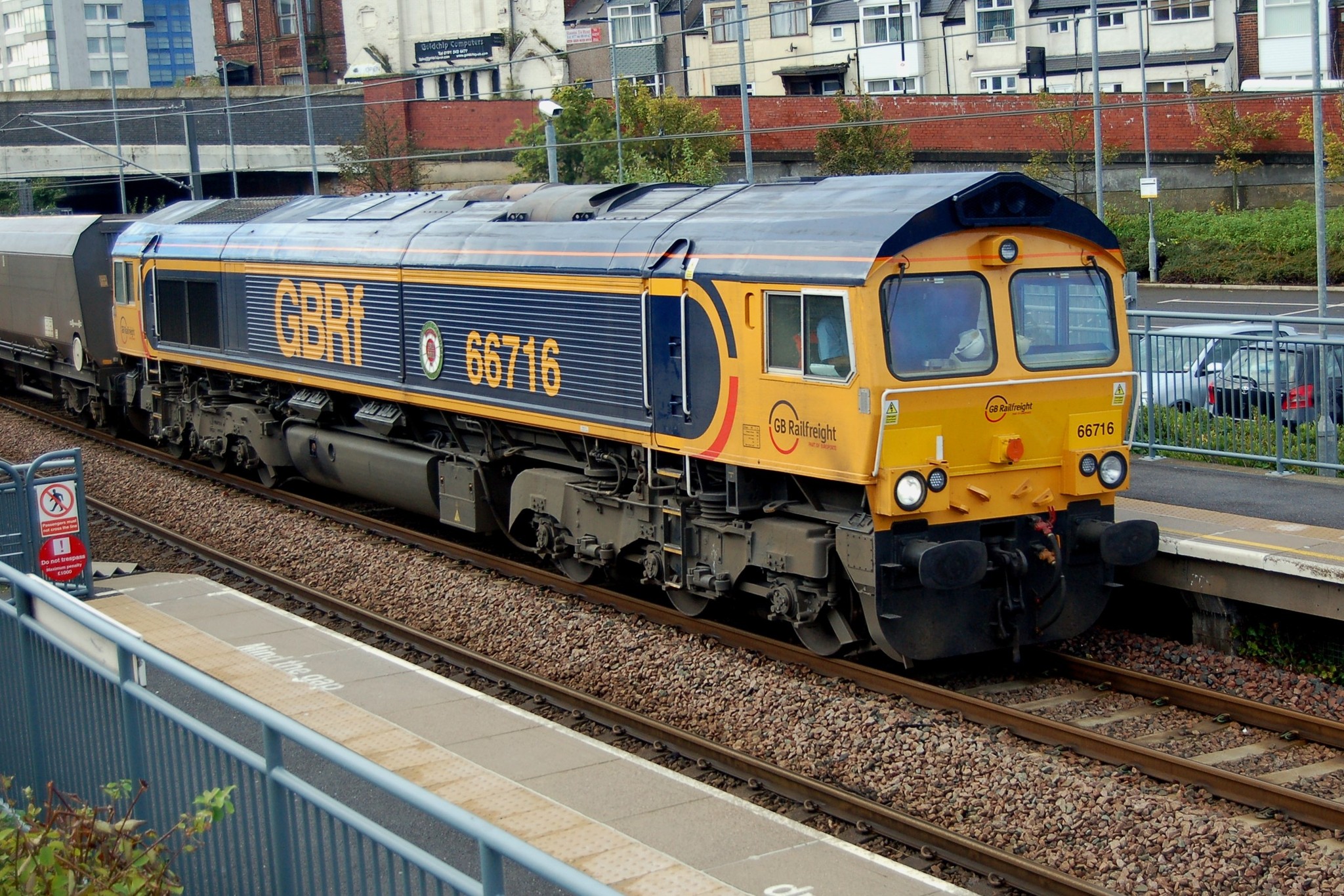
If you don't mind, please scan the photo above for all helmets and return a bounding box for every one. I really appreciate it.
[954,329,985,359]
[1016,334,1036,355]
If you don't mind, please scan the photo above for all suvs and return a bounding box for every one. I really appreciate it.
[1129,322,1298,415]
[1208,335,1344,435]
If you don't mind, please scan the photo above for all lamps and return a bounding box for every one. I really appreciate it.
[790,43,798,53]
[412,63,420,69]
[848,53,856,64]
[446,60,455,67]
[485,58,493,62]
[966,50,974,61]
[1211,65,1219,76]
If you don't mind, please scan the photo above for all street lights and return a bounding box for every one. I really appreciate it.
[214,54,238,199]
[107,20,156,215]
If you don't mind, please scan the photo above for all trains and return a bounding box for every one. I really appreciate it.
[0,172,1159,668]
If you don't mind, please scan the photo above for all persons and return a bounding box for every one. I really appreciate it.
[817,299,901,371]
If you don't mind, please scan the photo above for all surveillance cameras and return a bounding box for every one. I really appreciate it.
[537,99,565,119]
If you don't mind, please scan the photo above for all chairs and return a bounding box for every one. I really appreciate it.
[796,331,826,368]
[1222,340,1243,359]
[1183,341,1203,362]
